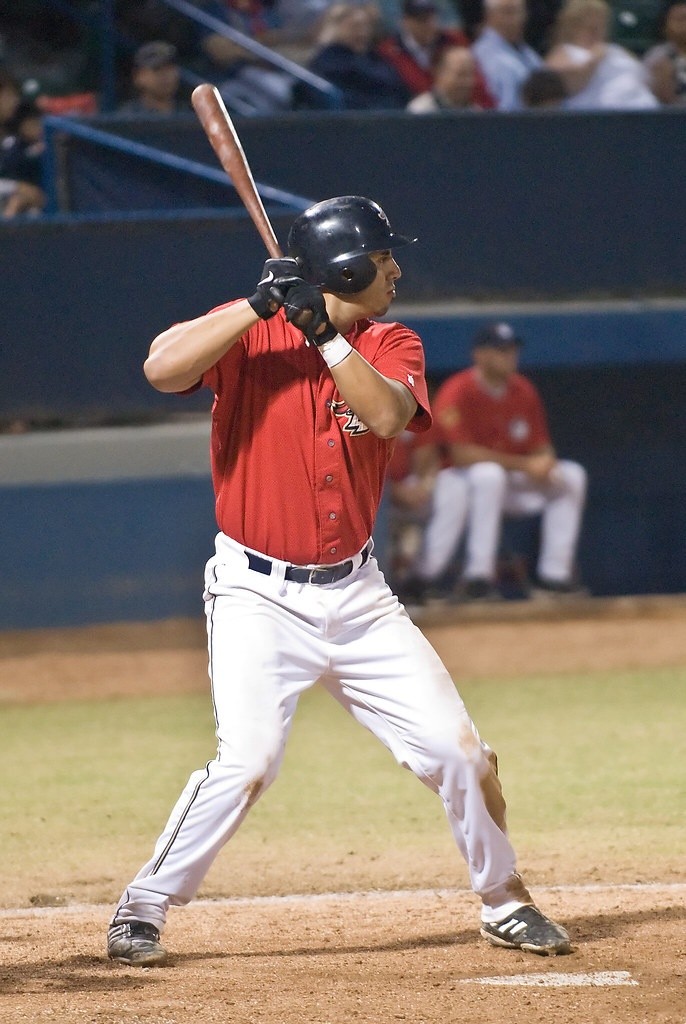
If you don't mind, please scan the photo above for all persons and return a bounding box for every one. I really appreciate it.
[638,0,686,106]
[377,0,496,109]
[407,45,477,112]
[107,196,571,964]
[470,0,542,108]
[384,324,587,599]
[96,0,248,113]
[546,0,661,108]
[0,69,43,214]
[294,2,411,109]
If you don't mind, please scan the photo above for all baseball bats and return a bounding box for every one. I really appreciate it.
[190,83,315,329]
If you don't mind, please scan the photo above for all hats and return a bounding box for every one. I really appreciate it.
[131,40,180,71]
[472,320,524,347]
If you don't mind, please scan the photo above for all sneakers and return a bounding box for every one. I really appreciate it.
[106,920,168,967]
[479,904,572,954]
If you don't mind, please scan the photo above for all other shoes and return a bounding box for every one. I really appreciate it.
[465,578,488,601]
[536,578,582,599]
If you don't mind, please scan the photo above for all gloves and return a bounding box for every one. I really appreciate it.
[283,284,352,368]
[247,255,303,322]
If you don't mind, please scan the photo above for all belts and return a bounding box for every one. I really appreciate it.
[244,547,369,585]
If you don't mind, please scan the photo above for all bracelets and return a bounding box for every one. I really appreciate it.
[317,333,352,368]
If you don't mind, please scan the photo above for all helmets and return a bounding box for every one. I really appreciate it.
[287,195,418,296]
